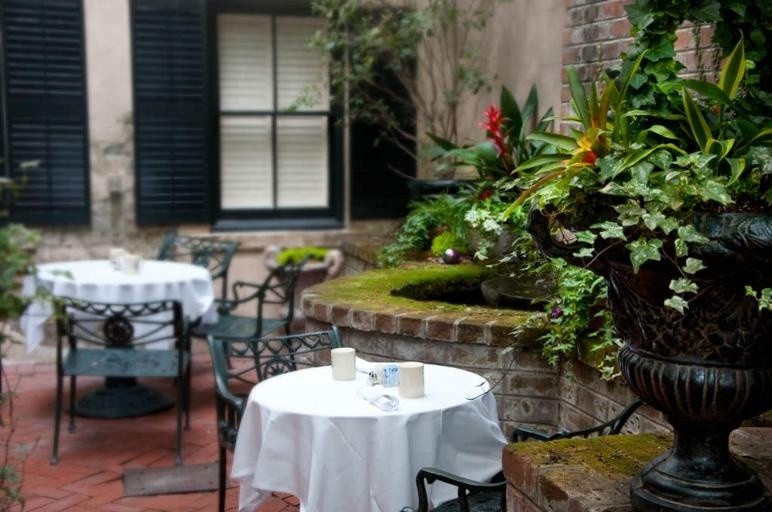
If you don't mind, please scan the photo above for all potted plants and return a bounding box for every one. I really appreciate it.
[262,246,345,332]
[498,28,772,512]
[288,0,498,229]
[424,84,559,304]
[378,189,470,268]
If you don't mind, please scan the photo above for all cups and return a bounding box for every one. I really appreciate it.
[331,348,356,379]
[112,249,126,270]
[398,361,425,398]
[125,255,139,275]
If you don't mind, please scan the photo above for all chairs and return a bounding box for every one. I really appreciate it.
[400,400,642,512]
[157,233,240,305]
[51,296,191,465]
[190,264,301,381]
[207,325,342,512]
